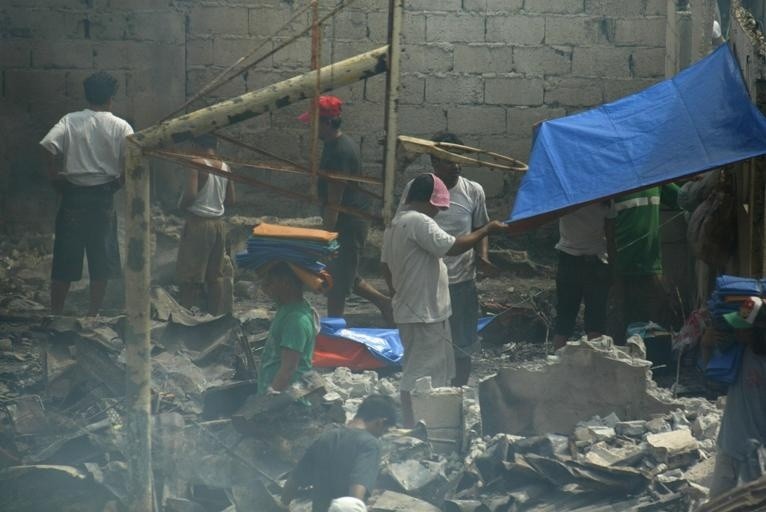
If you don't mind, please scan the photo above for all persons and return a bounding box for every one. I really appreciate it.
[299,97,396,330]
[276,395,397,512]
[36,68,135,318]
[249,263,321,496]
[170,134,237,316]
[554,167,733,353]
[700,322,766,497]
[380,134,510,427]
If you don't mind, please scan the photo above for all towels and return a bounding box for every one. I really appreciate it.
[426,173,451,212]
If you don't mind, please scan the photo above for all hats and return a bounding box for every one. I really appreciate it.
[297,95,343,124]
